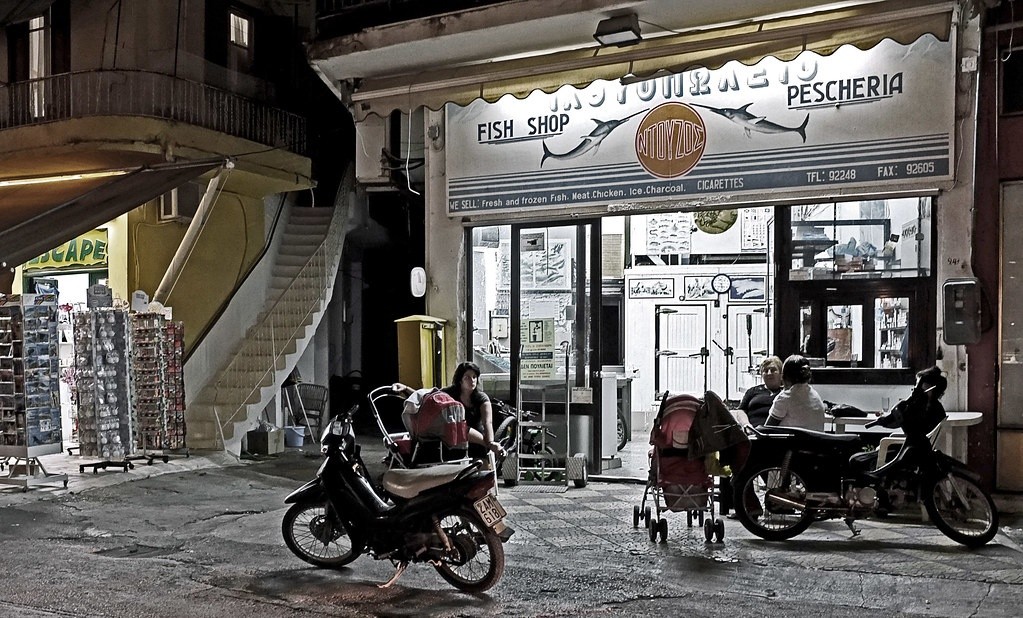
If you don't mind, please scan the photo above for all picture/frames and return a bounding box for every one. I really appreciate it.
[629,278,675,299]
[684,276,719,301]
[728,275,768,303]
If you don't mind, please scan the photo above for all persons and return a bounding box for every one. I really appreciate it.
[759,354,825,489]
[438,362,507,474]
[736,356,783,429]
[875,366,948,524]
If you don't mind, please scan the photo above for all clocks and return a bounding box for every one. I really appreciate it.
[711,273,732,294]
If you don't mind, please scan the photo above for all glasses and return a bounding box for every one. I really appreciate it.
[130,313,186,449]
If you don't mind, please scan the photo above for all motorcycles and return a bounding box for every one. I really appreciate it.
[729,364,1000,547]
[281,404,516,592]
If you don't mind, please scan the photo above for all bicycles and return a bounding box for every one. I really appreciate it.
[489,396,560,484]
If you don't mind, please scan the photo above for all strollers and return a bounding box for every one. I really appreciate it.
[365,386,473,470]
[633,389,726,543]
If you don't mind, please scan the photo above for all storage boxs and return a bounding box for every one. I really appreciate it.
[247,429,285,456]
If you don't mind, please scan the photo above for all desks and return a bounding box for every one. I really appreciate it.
[823,411,983,523]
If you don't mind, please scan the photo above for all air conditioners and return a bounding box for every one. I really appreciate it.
[161,180,199,223]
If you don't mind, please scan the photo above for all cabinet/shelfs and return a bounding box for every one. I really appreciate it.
[617,379,631,442]
[879,305,906,370]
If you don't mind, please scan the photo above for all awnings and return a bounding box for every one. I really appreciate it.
[0,158,234,272]
[351,8,962,126]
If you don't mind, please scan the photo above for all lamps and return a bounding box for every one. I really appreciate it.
[592,13,642,48]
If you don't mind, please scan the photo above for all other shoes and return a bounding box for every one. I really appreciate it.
[497,449,508,462]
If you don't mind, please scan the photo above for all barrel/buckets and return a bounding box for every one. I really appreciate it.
[284,426,305,447]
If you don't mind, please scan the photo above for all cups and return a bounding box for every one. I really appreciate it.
[882,397,889,412]
[850,354,858,368]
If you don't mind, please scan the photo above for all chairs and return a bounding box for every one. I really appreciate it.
[294,383,328,445]
